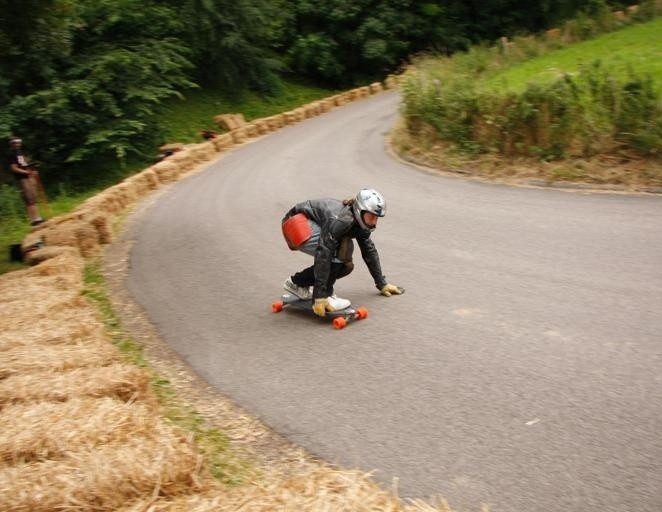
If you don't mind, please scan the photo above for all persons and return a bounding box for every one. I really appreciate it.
[279,186,404,321]
[8,134,44,226]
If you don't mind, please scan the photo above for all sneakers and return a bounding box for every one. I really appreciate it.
[283,276,313,301]
[312,295,351,311]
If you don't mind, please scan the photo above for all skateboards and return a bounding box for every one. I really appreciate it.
[272,293,368,330]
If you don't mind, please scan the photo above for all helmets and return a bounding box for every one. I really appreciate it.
[352,187,386,234]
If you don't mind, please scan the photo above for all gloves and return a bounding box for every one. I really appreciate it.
[381,283,405,297]
[312,298,334,317]
